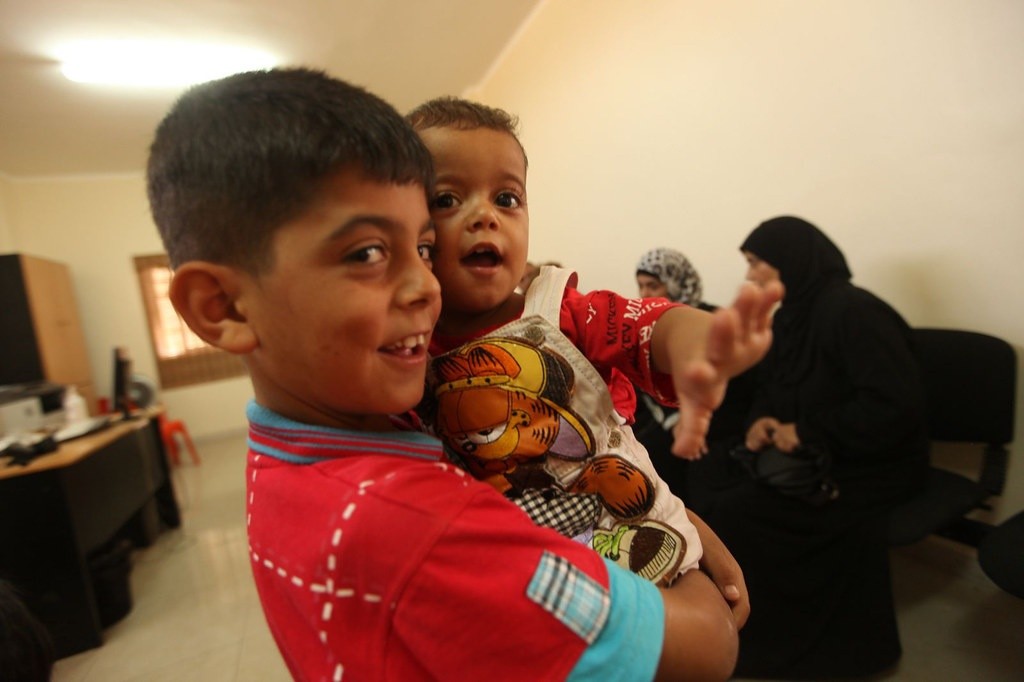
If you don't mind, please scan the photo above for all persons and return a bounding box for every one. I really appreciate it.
[147,66,753,682]
[626,217,925,682]
[400,98,785,584]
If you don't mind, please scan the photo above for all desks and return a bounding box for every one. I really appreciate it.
[0,406,172,645]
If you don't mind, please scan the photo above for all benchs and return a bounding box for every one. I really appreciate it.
[884,322,1024,602]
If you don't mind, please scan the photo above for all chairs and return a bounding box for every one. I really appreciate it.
[101,396,199,464]
